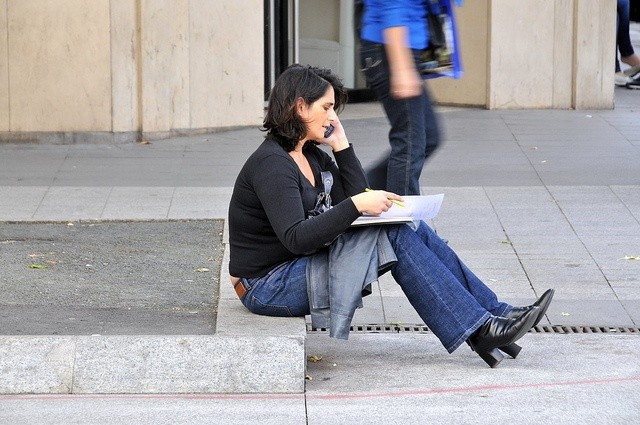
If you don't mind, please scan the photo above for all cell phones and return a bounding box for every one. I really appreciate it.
[324,124,334,138]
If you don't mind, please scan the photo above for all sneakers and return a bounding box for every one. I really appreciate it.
[615,73,634,87]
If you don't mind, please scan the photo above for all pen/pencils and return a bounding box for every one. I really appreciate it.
[365,188,406,206]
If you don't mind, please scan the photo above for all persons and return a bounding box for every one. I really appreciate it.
[615,0,640,89]
[228,63,556,368]
[347,1,466,195]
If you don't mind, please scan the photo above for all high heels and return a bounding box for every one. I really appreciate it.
[497,288,556,359]
[465,306,540,368]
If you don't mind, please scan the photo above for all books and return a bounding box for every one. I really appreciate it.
[349,194,417,228]
